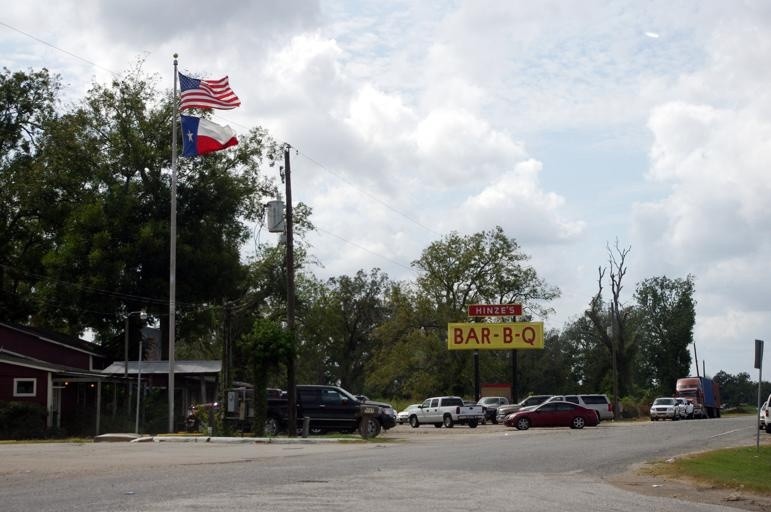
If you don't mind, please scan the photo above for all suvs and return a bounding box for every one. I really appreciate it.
[185,388,284,434]
[650,396,694,421]
[761,394,771,433]
[495,390,554,424]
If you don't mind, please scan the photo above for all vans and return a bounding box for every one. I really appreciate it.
[518,390,615,427]
[477,395,510,424]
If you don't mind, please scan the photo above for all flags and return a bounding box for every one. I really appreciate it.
[177,71,241,110]
[180,113,238,159]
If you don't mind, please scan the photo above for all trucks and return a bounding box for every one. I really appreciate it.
[676,376,722,419]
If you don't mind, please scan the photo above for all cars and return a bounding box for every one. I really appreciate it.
[502,400,601,430]
[397,401,425,425]
[756,400,766,429]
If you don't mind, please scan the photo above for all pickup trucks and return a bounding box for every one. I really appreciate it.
[262,384,398,437]
[407,395,485,428]
